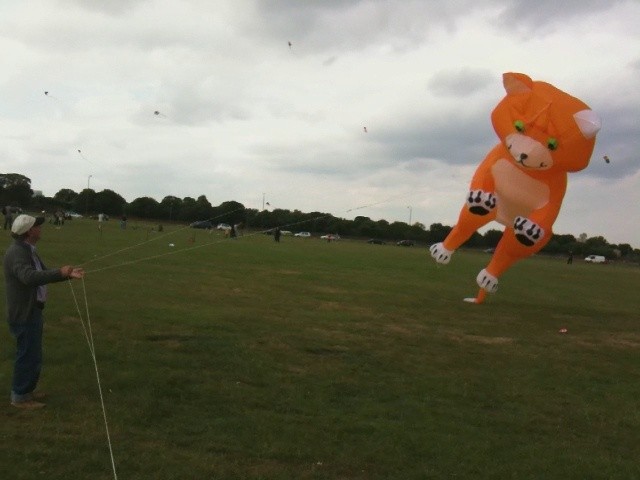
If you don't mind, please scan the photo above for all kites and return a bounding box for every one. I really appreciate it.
[428,71,602,305]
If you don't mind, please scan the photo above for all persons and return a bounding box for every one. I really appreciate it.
[121,214,130,229]
[230,223,239,238]
[327,233,333,244]
[63,213,66,222]
[98,209,106,231]
[67,214,71,223]
[158,224,164,233]
[2,202,19,231]
[3,214,84,409]
[567,249,574,263]
[274,225,283,240]
[39,208,46,218]
[52,211,59,226]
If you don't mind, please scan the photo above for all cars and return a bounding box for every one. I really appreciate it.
[367,239,387,245]
[321,234,341,240]
[89,214,109,220]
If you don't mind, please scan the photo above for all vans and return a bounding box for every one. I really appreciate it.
[584,255,605,263]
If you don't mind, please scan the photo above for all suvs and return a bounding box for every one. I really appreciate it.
[294,231,311,238]
[397,240,414,246]
[217,223,231,231]
[190,221,213,230]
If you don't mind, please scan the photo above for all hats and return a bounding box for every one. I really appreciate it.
[10,214,45,236]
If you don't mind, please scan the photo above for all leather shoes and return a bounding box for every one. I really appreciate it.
[11,400,48,409]
